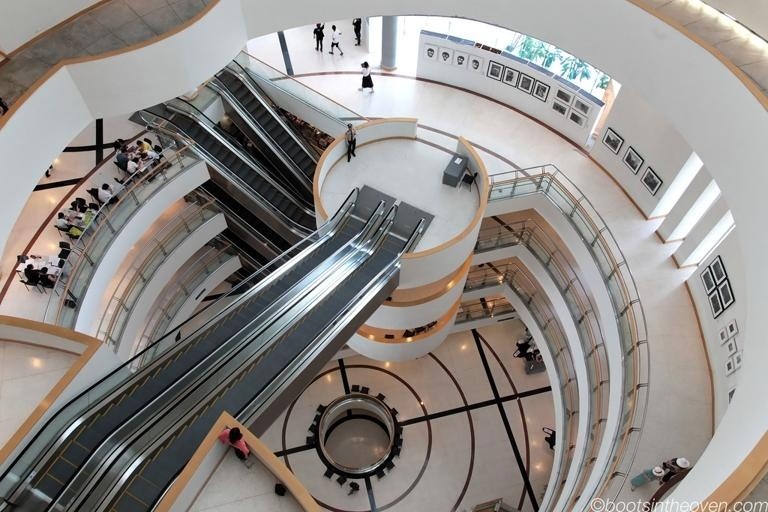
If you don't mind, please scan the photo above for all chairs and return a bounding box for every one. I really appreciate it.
[16,138,172,297]
[459,172,477,192]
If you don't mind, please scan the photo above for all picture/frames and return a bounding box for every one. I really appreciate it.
[717,319,742,376]
[727,384,736,403]
[700,255,735,319]
[485,60,550,103]
[602,127,664,198]
[550,86,592,129]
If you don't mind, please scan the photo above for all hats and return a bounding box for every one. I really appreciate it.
[652,467,665,477]
[677,457,690,469]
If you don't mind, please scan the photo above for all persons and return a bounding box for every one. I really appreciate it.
[660,457,690,485]
[359,61,375,94]
[352,18,361,46]
[312,23,325,53]
[216,424,250,462]
[39,267,57,287]
[114,139,127,150]
[346,123,358,162]
[629,466,663,493]
[140,150,160,163]
[116,149,128,165]
[135,140,153,153]
[55,212,69,230]
[280,107,335,151]
[97,184,114,205]
[126,157,142,178]
[24,264,40,283]
[328,25,343,56]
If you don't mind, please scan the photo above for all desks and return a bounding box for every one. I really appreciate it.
[443,152,468,188]
